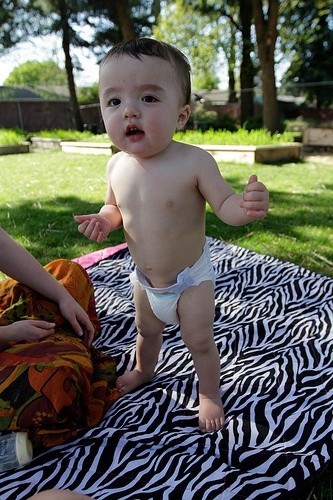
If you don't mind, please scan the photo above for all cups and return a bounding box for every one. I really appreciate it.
[0,432,32,471]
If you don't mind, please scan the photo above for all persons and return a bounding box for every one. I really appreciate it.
[0,226,95,346]
[27,488,95,500]
[73,37,269,432]
